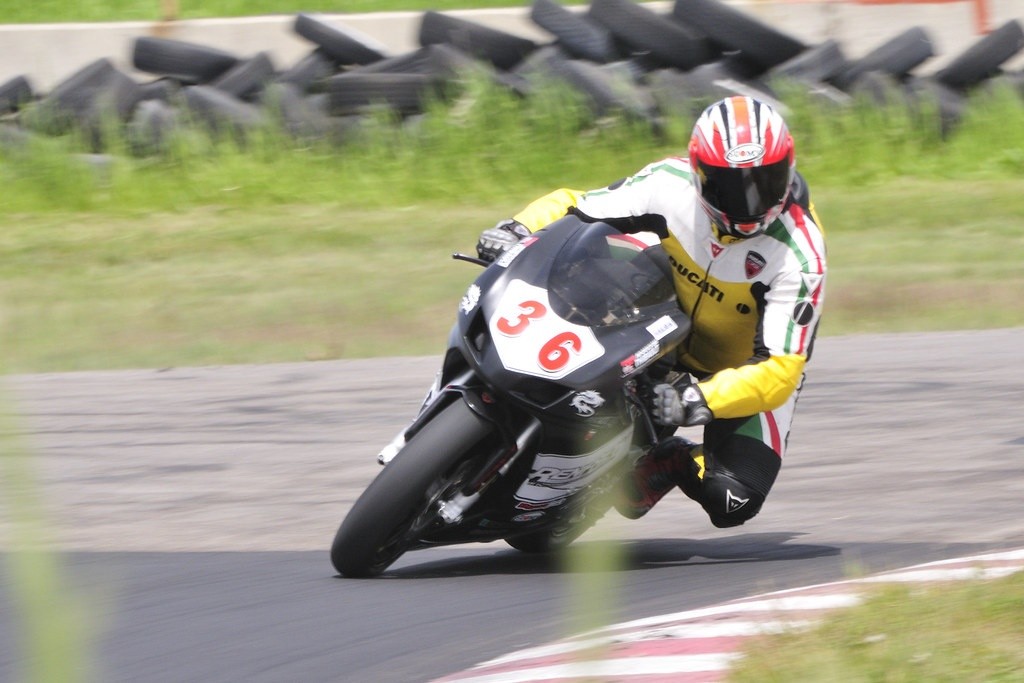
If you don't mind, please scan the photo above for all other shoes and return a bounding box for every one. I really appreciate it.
[616,434,692,521]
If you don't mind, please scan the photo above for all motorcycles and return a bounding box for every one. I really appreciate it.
[329,214,703,580]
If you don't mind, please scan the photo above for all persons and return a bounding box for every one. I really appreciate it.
[443,95,827,529]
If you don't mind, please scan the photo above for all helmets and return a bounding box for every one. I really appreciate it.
[687,94,798,239]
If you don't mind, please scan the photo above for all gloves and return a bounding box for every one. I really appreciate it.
[476,218,531,265]
[649,382,717,430]
